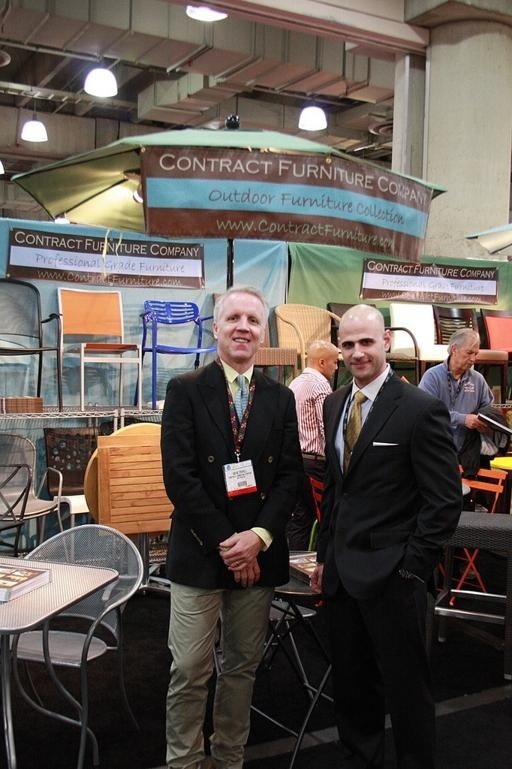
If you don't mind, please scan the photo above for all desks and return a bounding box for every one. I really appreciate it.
[250,549,332,769]
[425,513,512,680]
[84,423,174,612]
[0,556,121,769]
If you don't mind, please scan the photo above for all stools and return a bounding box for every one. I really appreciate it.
[213,293,296,378]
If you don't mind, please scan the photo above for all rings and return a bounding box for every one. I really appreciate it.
[236,561,240,566]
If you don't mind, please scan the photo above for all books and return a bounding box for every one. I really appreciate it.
[481,412,512,438]
[0,562,52,604]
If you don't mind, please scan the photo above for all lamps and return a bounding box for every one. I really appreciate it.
[298,95,328,131]
[186,6,228,22]
[83,58,118,97]
[21,95,49,142]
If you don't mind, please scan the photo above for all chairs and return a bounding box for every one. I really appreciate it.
[0,277,64,412]
[134,300,217,410]
[0,464,33,557]
[436,464,506,606]
[275,304,342,384]
[328,302,419,391]
[0,432,68,562]
[9,524,144,733]
[57,287,142,411]
[308,478,324,552]
[389,304,474,382]
[432,305,507,403]
[480,308,512,378]
[43,426,99,563]
[263,600,316,707]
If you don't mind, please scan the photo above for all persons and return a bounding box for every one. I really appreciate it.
[282,340,339,551]
[160,285,305,769]
[311,304,463,767]
[420,327,496,483]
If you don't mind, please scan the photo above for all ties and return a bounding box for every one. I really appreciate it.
[233,376,250,423]
[343,391,371,479]
